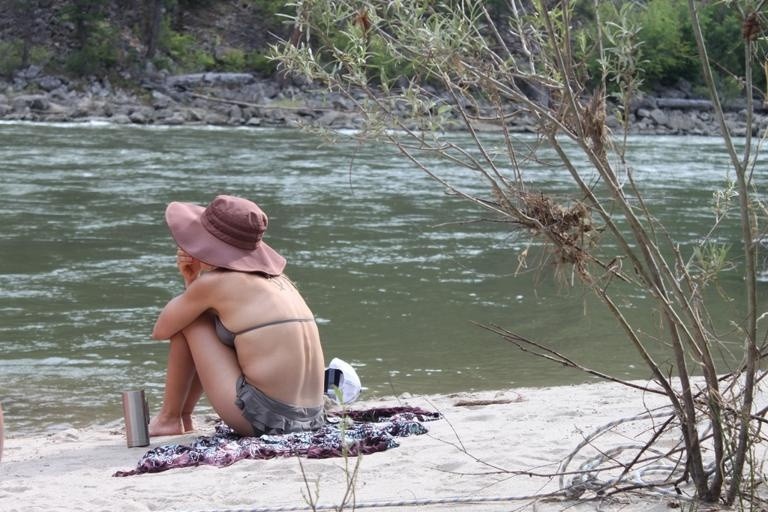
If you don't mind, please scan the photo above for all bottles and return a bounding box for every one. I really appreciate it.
[122,389,149,449]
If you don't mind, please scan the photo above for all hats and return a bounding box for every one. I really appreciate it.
[164,194,287,275]
[325,357,363,405]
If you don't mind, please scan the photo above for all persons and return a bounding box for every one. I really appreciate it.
[148,194,326,439]
